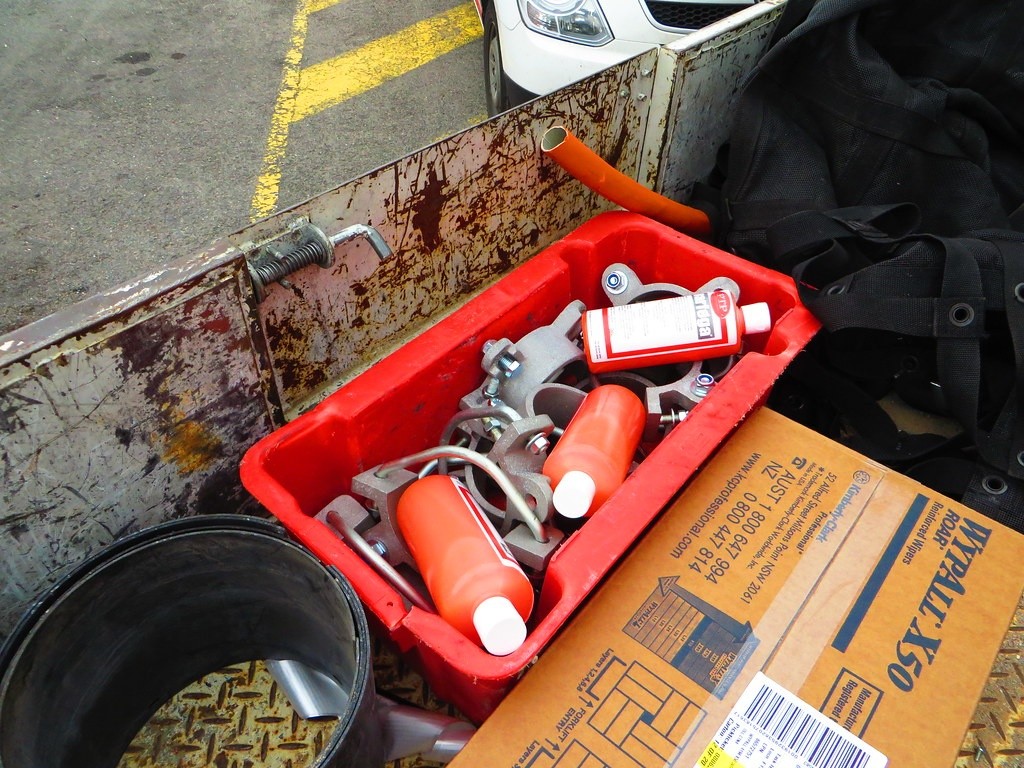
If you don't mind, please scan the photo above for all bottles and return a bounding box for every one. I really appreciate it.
[542,385,646,517]
[395,475,533,656]
[582,293,772,373]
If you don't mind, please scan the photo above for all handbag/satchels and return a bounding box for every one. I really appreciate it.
[689,1,1023,528]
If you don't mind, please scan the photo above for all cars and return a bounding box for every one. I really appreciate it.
[473,0,767,119]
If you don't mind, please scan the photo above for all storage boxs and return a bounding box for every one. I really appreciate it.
[443,405,1023,765]
[241,216,823,723]
